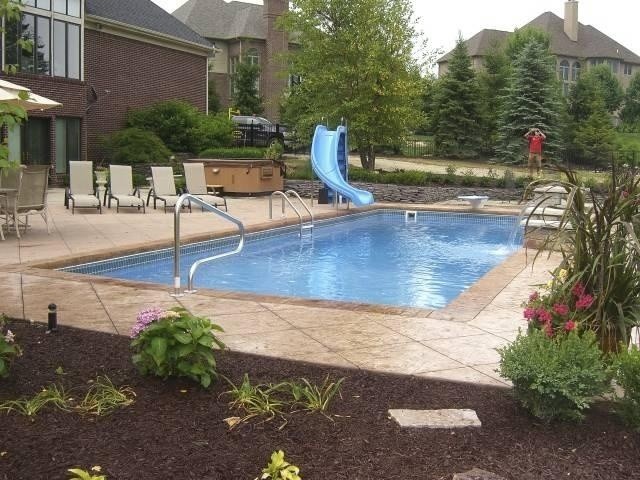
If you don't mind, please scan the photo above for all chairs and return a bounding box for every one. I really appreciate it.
[103,165,145,213]
[183,163,227,212]
[146,167,191,214]
[64,161,101,214]
[0,165,26,232]
[9,164,54,235]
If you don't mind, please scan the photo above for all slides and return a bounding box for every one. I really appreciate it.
[311,125,374,207]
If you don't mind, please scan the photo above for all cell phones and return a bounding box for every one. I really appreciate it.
[533,128,538,132]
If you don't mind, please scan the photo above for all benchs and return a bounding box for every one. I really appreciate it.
[520,219,577,251]
[525,207,588,220]
[531,187,583,205]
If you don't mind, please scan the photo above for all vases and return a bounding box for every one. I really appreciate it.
[597,325,635,356]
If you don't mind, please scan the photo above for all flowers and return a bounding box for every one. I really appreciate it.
[518,150,640,359]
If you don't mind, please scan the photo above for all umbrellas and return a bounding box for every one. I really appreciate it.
[1,79,32,91]
[0,86,62,115]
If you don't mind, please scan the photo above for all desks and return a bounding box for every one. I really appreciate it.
[0,188,20,240]
[206,185,224,197]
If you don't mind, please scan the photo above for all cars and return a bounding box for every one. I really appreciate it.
[231,116,294,149]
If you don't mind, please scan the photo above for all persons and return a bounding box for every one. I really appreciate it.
[524,128,546,176]
